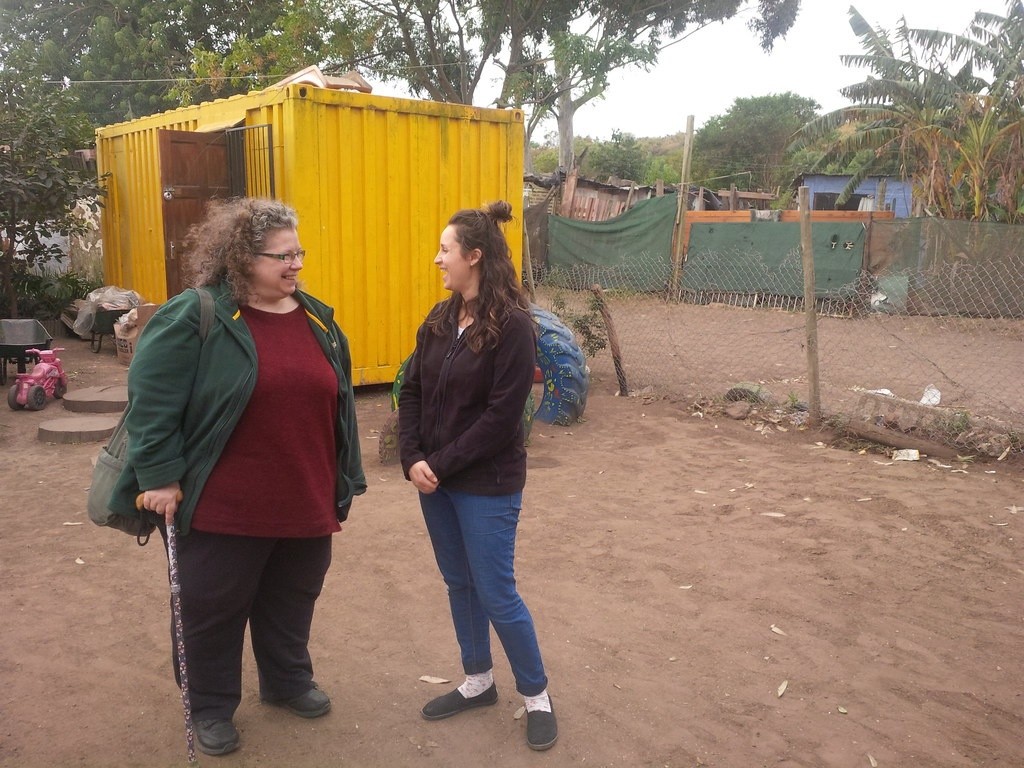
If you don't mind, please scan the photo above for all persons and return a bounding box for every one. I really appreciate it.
[125,197,367,757]
[396,200,558,751]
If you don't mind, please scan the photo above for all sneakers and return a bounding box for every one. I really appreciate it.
[259,687,331,718]
[195,719,240,754]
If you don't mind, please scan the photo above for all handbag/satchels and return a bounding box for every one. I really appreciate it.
[88,287,215,546]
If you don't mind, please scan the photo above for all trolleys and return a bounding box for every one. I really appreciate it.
[70,304,131,353]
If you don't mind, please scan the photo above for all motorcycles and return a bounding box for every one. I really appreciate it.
[8,348,67,411]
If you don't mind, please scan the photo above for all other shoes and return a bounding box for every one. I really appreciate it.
[526,693,557,750]
[421,680,498,720]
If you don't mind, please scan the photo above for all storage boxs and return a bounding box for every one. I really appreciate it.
[113,304,161,366]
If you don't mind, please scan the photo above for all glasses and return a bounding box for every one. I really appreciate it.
[253,250,305,263]
